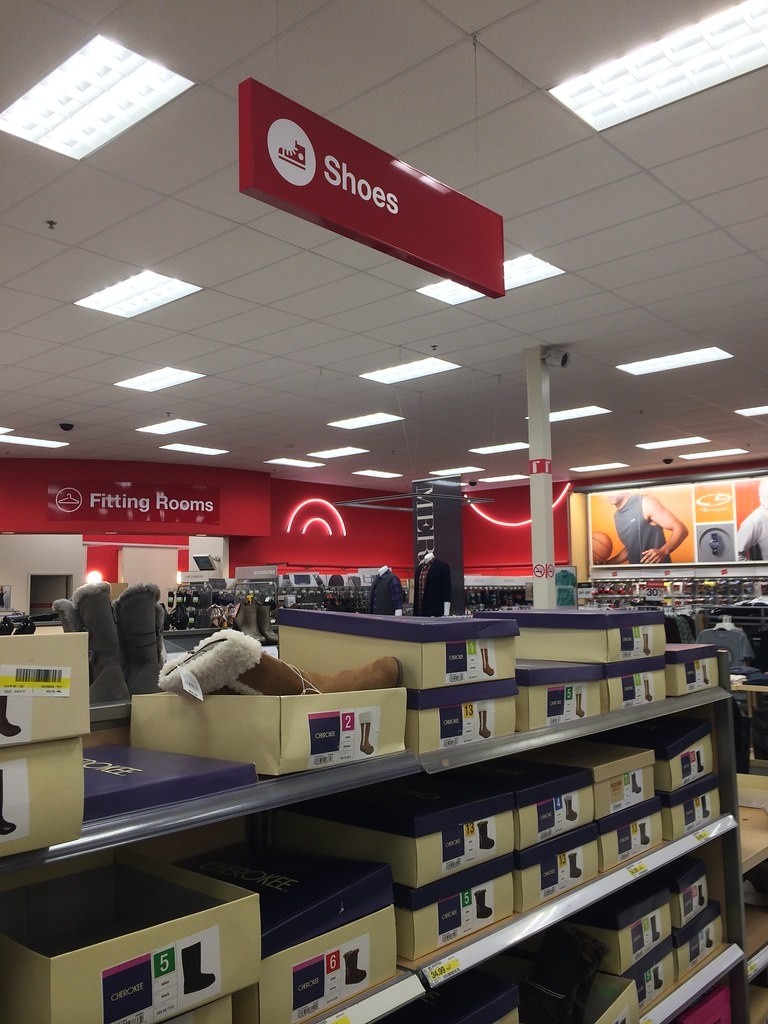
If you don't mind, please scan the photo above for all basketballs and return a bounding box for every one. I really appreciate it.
[593,531,613,563]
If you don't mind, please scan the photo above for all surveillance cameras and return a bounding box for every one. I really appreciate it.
[60,423,74,431]
[468,480,477,486]
[663,459,673,464]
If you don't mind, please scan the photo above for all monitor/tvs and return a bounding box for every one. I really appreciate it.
[193,554,216,571]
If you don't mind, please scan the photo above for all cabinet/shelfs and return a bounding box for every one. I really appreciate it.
[739,806,768,1024]
[0,649,747,1024]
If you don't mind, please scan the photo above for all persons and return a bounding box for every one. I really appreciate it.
[737,474,768,561]
[595,489,690,565]
[367,565,403,616]
[414,552,452,617]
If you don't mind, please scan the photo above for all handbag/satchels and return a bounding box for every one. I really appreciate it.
[171,602,189,630]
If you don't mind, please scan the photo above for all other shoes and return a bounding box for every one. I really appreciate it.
[14,617,36,635]
[0,615,14,635]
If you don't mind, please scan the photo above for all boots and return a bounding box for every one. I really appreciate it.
[159,630,404,697]
[477,821,494,850]
[477,702,491,738]
[0,769,16,835]
[646,885,714,993]
[575,685,586,718]
[474,889,492,919]
[254,603,279,645]
[0,696,21,737]
[343,949,367,985]
[639,823,650,846]
[234,605,266,644]
[568,853,582,878]
[479,640,495,676]
[181,941,216,994]
[640,628,710,701]
[110,583,166,696]
[359,711,374,755]
[632,773,642,794]
[564,796,577,821]
[52,581,130,703]
[696,750,704,773]
[701,796,710,818]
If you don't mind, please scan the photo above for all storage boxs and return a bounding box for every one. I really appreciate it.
[2,604,734,1023]
